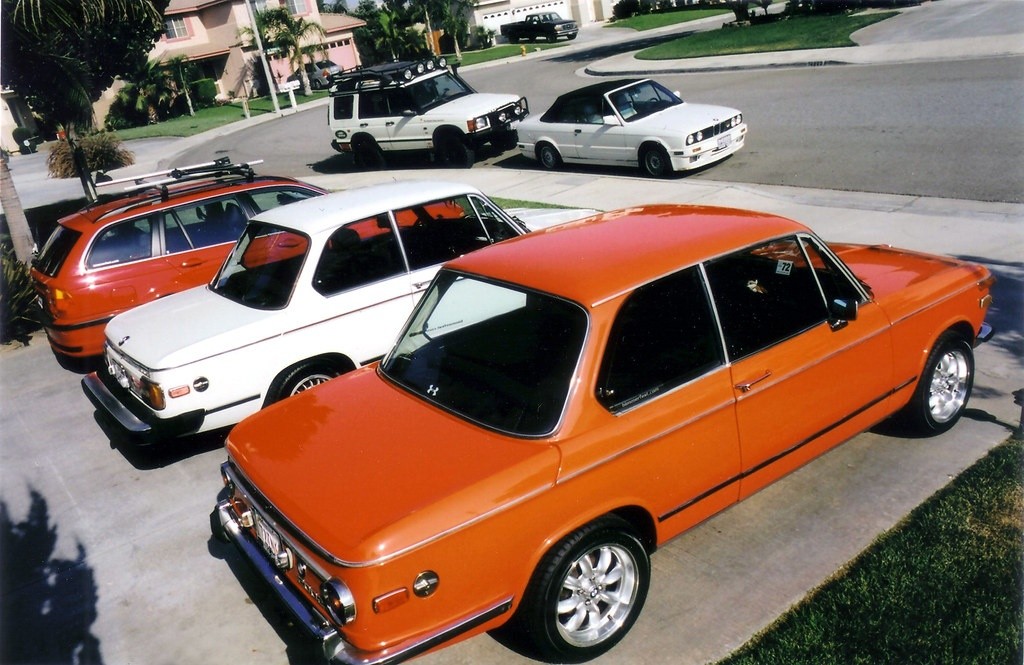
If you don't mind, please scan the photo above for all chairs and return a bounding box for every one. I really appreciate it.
[333,228,373,287]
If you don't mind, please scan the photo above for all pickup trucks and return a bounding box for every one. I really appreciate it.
[499,11,579,45]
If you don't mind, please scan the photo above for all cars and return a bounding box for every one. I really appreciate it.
[209,201,998,665]
[30,158,465,359]
[517,77,749,176]
[287,58,345,90]
[80,172,607,449]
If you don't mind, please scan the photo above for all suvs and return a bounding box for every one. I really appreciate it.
[325,54,530,167]
